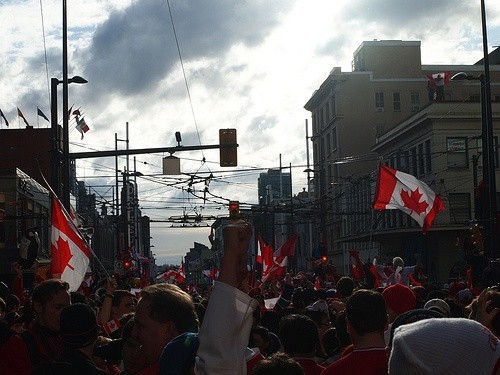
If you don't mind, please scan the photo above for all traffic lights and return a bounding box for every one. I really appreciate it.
[123,257,130,275]
[470,227,481,248]
[322,256,328,271]
[228,201,239,220]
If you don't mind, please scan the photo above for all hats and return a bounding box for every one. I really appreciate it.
[388,317,500,375]
[158,332,199,375]
[448,282,460,292]
[424,299,451,314]
[382,283,417,314]
[59,303,101,349]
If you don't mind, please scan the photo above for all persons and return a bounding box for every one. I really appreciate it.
[1,259,209,375]
[192,222,258,375]
[433,74,444,100]
[246,249,500,375]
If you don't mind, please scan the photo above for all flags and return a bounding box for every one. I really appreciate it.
[38,108,49,122]
[395,270,402,285]
[0,109,9,126]
[351,254,362,279]
[373,164,443,234]
[203,267,220,280]
[257,232,298,282]
[18,109,29,126]
[294,274,305,280]
[68,107,89,140]
[428,72,449,86]
[49,197,91,292]
[103,318,122,335]
[177,263,186,282]
[370,258,381,287]
[467,269,472,290]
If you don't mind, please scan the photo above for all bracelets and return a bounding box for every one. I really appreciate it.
[105,293,112,298]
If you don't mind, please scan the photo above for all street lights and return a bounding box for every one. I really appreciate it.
[451,72,492,260]
[303,168,328,283]
[50,76,90,198]
[122,172,145,282]
